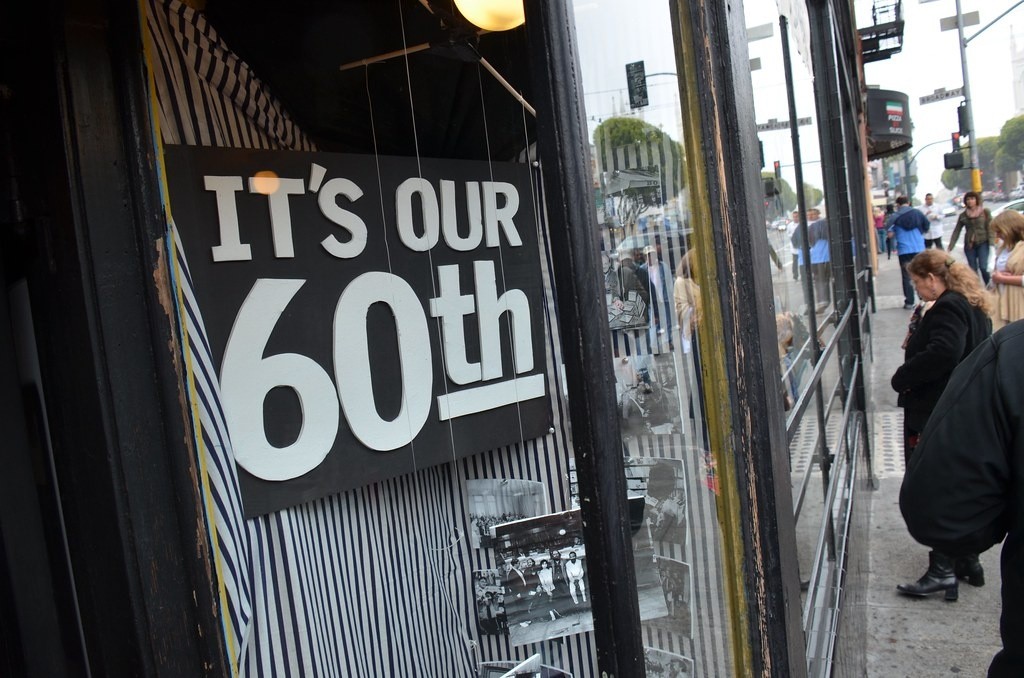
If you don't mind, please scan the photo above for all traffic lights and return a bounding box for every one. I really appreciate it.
[952,130,960,152]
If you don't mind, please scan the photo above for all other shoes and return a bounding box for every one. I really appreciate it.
[669,343,674,351]
[904,302,916,309]
[574,599,578,604]
[583,598,587,602]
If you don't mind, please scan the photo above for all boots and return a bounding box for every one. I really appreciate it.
[896,550,958,600]
[953,550,986,586]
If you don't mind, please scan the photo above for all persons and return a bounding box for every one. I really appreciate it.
[918,193,946,250]
[873,204,898,253]
[946,192,992,285]
[787,210,832,315]
[659,568,684,618]
[469,512,528,548]
[899,319,1024,678]
[991,210,1024,325]
[890,250,998,602]
[647,464,680,542]
[476,570,506,632]
[600,234,793,450]
[887,195,930,309]
[509,550,588,627]
[671,660,689,678]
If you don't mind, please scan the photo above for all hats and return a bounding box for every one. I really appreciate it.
[619,254,633,262]
[643,245,656,254]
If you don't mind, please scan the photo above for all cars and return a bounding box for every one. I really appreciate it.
[991,199,1024,220]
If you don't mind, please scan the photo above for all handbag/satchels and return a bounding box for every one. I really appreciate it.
[987,246,998,272]
